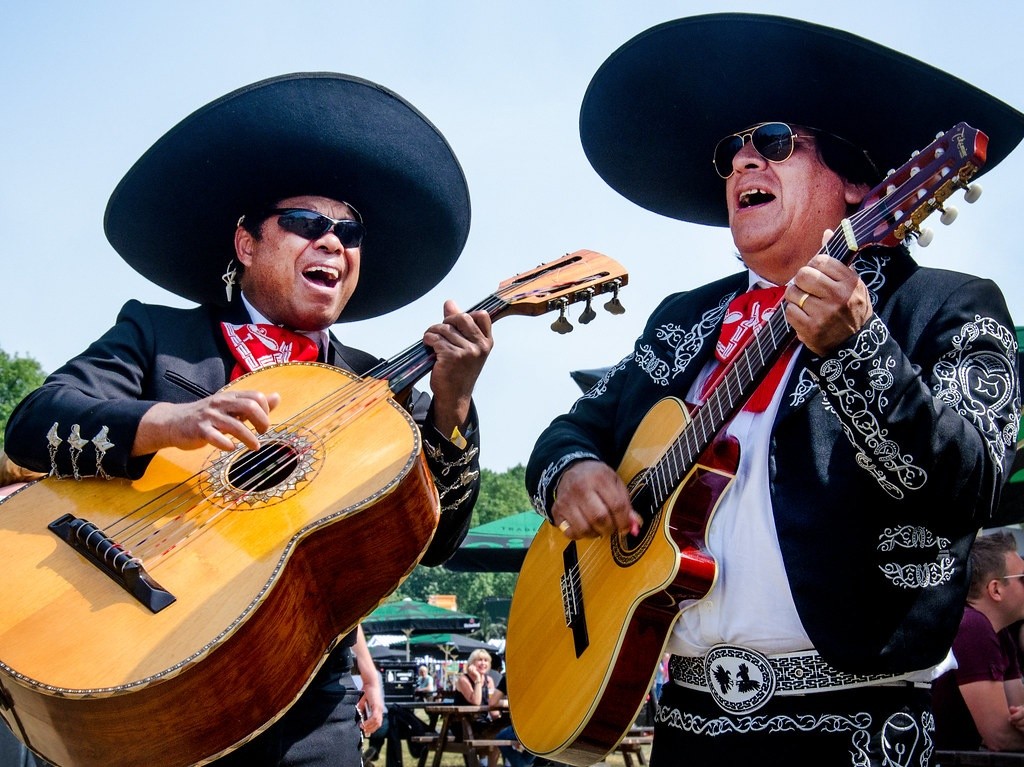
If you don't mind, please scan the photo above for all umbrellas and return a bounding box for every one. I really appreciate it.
[442,507,545,573]
[361,598,481,662]
[388,633,500,690]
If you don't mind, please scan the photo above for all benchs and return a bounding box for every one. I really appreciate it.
[461,738,650,766]
[411,736,462,767]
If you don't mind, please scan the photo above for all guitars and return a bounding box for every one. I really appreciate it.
[1,248,630,767]
[504,118,991,767]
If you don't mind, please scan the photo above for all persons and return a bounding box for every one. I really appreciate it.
[525,14,1024,766]
[0,455,1023,767]
[0,72,494,767]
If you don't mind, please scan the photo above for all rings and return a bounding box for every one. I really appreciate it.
[557,520,570,532]
[799,293,810,309]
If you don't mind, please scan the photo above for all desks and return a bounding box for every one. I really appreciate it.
[424,704,494,767]
[374,701,452,767]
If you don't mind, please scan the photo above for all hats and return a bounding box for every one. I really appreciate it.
[580,13,1024,228]
[103,74,471,324]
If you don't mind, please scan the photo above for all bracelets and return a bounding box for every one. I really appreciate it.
[474,679,482,684]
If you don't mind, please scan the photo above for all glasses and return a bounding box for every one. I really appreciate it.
[1004,574,1024,585]
[270,207,363,249]
[713,122,814,179]
